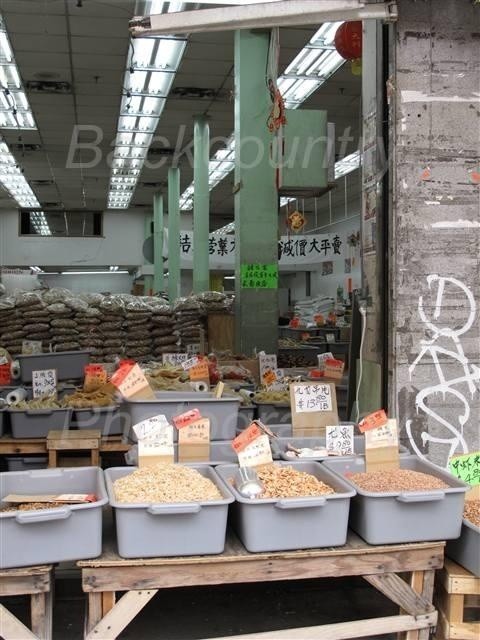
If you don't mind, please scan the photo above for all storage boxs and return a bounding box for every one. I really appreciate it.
[278,325,351,419]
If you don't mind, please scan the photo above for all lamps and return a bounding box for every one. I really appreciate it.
[175,134,236,211]
[0,142,44,208]
[106,1,205,210]
[29,211,53,236]
[1,17,40,131]
[273,22,364,110]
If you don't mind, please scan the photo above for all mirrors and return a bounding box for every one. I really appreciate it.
[19,208,102,238]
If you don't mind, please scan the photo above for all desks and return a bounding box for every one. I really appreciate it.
[78,531,443,640]
[0,564,61,639]
[434,560,480,640]
